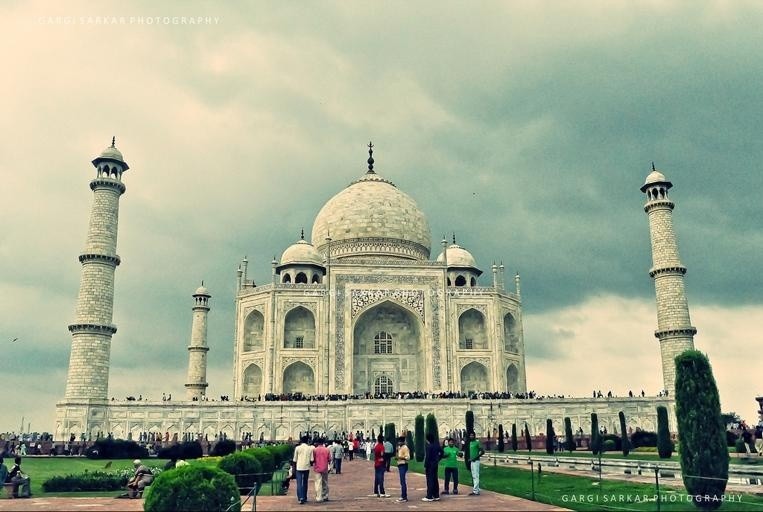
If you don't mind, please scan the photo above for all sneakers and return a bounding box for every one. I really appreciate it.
[373,490,481,503]
[299,496,329,505]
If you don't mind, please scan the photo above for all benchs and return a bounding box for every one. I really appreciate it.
[2,482,21,498]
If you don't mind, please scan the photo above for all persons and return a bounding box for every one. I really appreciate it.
[738,428,752,456]
[728,419,762,430]
[441,438,464,495]
[420,434,444,503]
[467,432,485,497]
[574,426,617,438]
[0,456,9,494]
[4,457,30,500]
[279,455,394,505]
[393,437,410,503]
[106,386,668,401]
[553,435,565,451]
[752,425,763,457]
[115,459,156,500]
